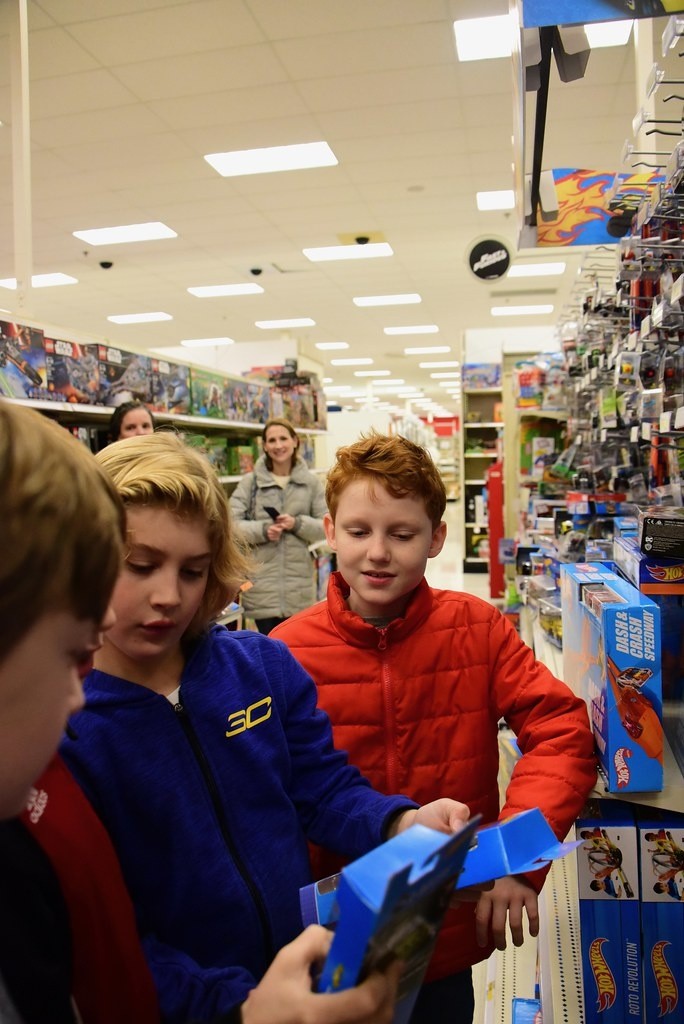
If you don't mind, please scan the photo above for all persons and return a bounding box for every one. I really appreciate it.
[0,398,162,1024]
[225,418,334,636]
[55,429,471,1022]
[266,435,600,1024]
[100,399,155,450]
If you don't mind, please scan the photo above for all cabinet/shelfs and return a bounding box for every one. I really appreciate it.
[0,312,337,642]
[459,363,506,575]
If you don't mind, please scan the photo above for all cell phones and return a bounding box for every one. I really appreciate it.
[263,506,280,520]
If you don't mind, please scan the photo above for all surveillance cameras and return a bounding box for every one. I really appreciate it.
[100,262,112,269]
[356,238,369,244]
[251,269,262,275]
